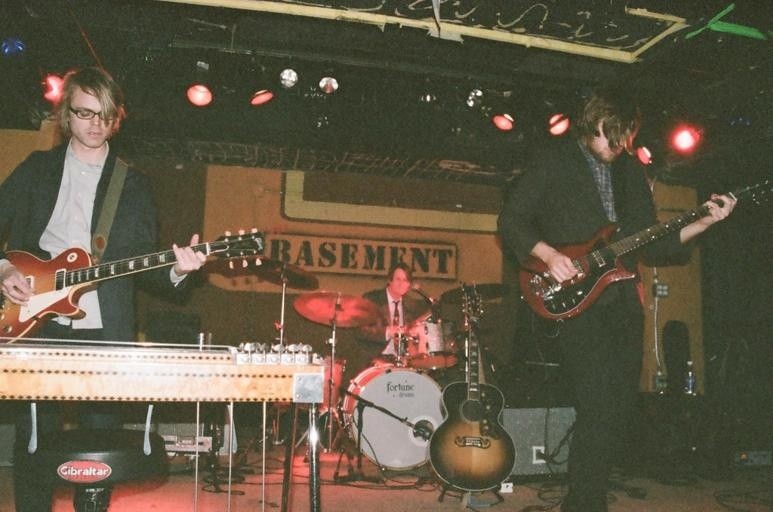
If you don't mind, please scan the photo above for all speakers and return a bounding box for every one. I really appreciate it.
[499,406,577,484]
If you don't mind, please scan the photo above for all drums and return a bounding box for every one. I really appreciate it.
[340,362,446,472]
[405,321,459,372]
[27,427,165,484]
[296,355,346,415]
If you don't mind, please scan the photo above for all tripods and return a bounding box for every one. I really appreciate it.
[296,337,345,454]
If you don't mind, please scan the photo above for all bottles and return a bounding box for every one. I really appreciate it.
[685,361,696,395]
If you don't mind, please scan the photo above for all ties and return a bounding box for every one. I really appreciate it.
[393,301,399,353]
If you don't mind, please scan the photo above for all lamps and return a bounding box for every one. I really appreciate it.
[163,46,342,111]
[418,78,703,165]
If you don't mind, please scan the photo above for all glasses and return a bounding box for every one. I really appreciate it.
[69,106,112,120]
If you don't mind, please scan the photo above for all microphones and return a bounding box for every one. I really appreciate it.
[411,284,432,303]
[412,424,432,440]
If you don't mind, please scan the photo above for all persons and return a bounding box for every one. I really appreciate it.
[0,69,207,511]
[497,82,739,487]
[335,262,432,423]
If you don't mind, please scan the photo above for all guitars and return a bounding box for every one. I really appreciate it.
[431,280,514,492]
[518,180,773,323]
[0,228,266,343]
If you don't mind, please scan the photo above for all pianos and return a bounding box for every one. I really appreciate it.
[0,341,326,405]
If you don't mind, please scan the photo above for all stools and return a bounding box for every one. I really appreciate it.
[26,426,169,512]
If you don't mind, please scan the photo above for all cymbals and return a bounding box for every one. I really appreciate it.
[293,289,378,329]
[251,256,319,292]
[443,282,510,302]
